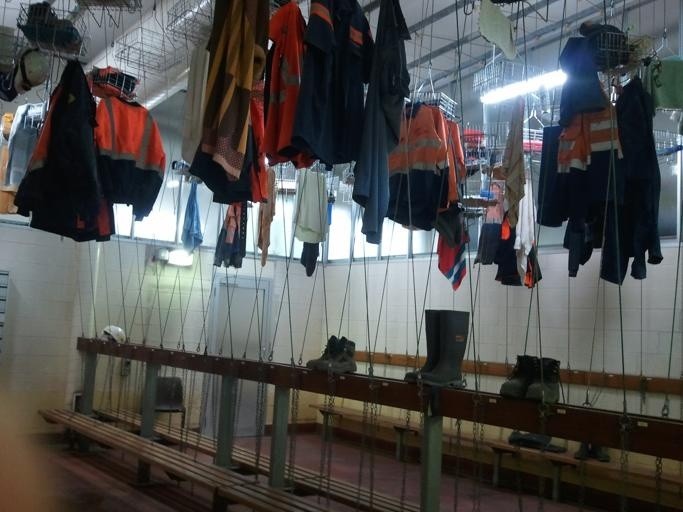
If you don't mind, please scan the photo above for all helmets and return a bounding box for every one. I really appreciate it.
[14,46,48,94]
[103,326,126,344]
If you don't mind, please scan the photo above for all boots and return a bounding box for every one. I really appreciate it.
[404,310,469,388]
[307,336,356,373]
[500,356,560,403]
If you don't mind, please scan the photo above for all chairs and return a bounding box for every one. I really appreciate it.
[153,376,187,452]
